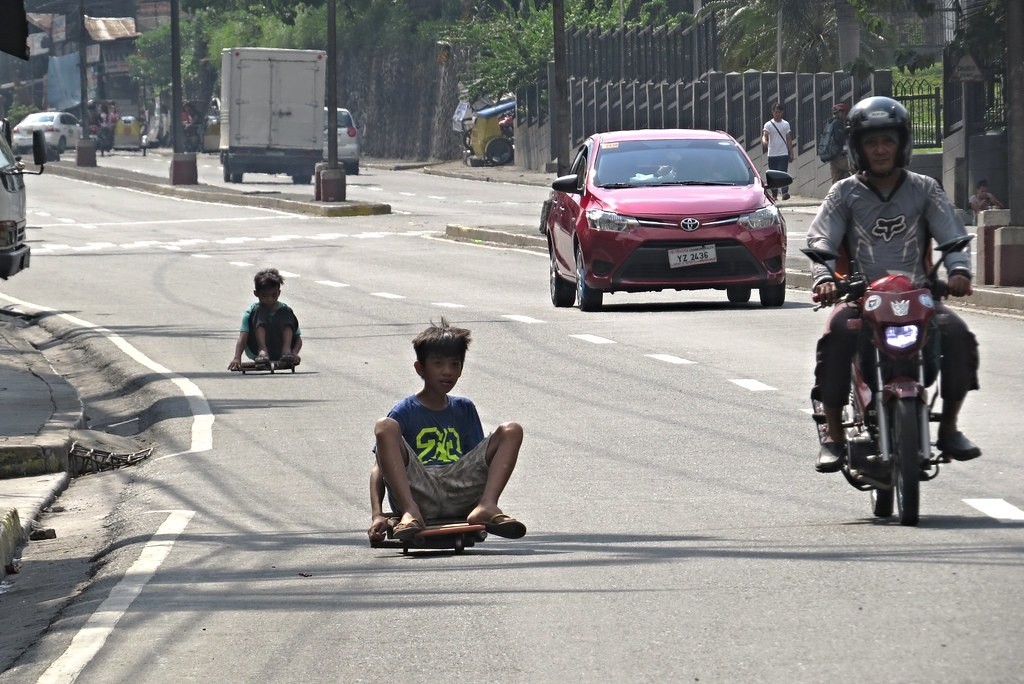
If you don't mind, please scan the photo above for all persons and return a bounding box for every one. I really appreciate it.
[228,268,302,370]
[830,104,851,183]
[368,315,526,540]
[969,181,1003,213]
[88,100,117,149]
[807,97,981,474]
[186,111,199,134]
[762,103,794,200]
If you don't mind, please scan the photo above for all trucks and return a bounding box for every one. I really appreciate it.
[0,125,45,276]
[219,48,326,186]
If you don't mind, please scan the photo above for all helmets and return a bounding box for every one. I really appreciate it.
[846,96,913,171]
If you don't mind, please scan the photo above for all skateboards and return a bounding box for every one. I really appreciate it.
[240,356,297,374]
[391,522,489,557]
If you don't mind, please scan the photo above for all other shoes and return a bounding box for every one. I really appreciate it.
[782,192,790,200]
[772,196,777,200]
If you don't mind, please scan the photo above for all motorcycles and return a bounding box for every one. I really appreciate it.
[802,234,980,521]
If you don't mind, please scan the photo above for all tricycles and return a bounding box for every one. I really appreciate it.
[87,99,118,154]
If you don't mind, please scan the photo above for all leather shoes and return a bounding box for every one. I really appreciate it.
[936,432,982,462]
[815,442,845,473]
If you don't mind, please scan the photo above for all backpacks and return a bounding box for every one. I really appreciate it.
[818,117,842,162]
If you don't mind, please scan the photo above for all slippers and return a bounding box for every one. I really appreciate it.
[393,518,424,538]
[254,356,270,365]
[279,352,295,362]
[476,514,526,539]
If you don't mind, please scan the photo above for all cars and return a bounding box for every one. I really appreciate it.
[7,111,83,152]
[323,108,362,174]
[538,129,796,312]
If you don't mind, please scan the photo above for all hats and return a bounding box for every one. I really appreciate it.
[832,103,848,113]
